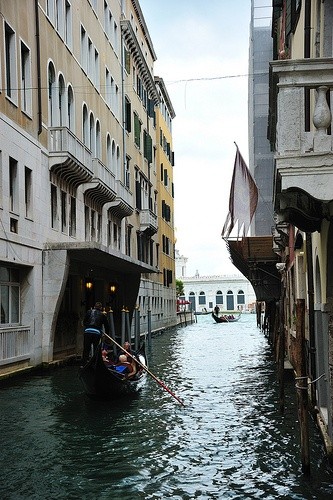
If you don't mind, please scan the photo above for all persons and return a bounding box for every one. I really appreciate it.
[82,301,111,365]
[222,315,235,319]
[215,304,220,317]
[117,340,142,377]
[114,354,134,379]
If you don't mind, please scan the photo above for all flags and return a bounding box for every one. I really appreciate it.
[222,149,258,242]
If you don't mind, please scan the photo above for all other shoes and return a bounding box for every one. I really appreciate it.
[80,364,85,368]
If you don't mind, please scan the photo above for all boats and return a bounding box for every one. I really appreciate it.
[212,310,242,323]
[78,328,149,400]
